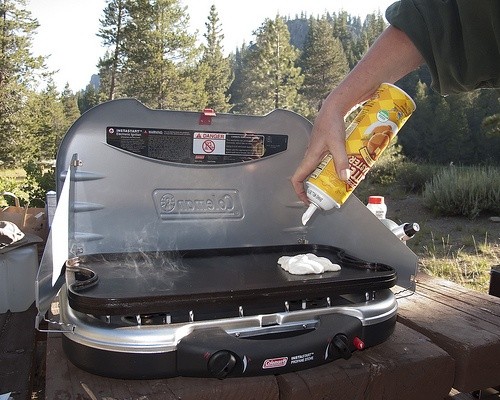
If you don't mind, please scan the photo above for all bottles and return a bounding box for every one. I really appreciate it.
[391,222,420,242]
[300,83,417,226]
[367,195,387,219]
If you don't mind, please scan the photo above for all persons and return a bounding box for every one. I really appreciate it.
[292,0,500,207]
